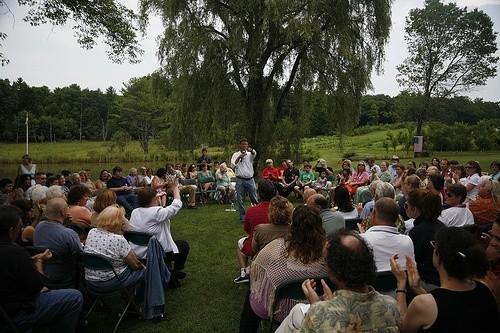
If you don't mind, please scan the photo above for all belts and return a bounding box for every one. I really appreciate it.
[236,175,254,181]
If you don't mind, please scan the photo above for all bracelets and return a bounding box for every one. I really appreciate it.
[395,289,407,292]
[35,259,43,263]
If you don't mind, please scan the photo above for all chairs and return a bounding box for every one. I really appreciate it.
[0,171,218,333]
[268,168,500,333]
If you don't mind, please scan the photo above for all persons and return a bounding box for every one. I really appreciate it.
[0,147,242,227]
[436,184,475,227]
[34,198,84,289]
[89,189,128,231]
[81,204,146,319]
[403,189,449,284]
[239,205,334,333]
[233,178,278,283]
[260,154,500,218]
[360,181,395,218]
[127,175,190,280]
[480,218,500,301]
[389,226,500,333]
[230,138,260,222]
[306,194,345,236]
[0,203,82,333]
[353,197,416,292]
[331,185,359,219]
[250,195,297,261]
[469,179,498,224]
[10,198,46,246]
[64,184,92,225]
[273,228,403,333]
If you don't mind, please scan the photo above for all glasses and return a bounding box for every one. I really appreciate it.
[358,164,364,166]
[405,203,415,210]
[267,162,272,164]
[370,207,376,212]
[465,166,475,169]
[444,193,454,197]
[429,240,437,251]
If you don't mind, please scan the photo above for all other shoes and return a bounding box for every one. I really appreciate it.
[240,218,244,221]
[168,268,186,279]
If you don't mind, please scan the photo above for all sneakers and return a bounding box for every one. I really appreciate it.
[234,274,250,283]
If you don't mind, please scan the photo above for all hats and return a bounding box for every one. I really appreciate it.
[266,159,273,164]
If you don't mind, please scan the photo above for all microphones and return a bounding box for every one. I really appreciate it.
[240,151,245,162]
